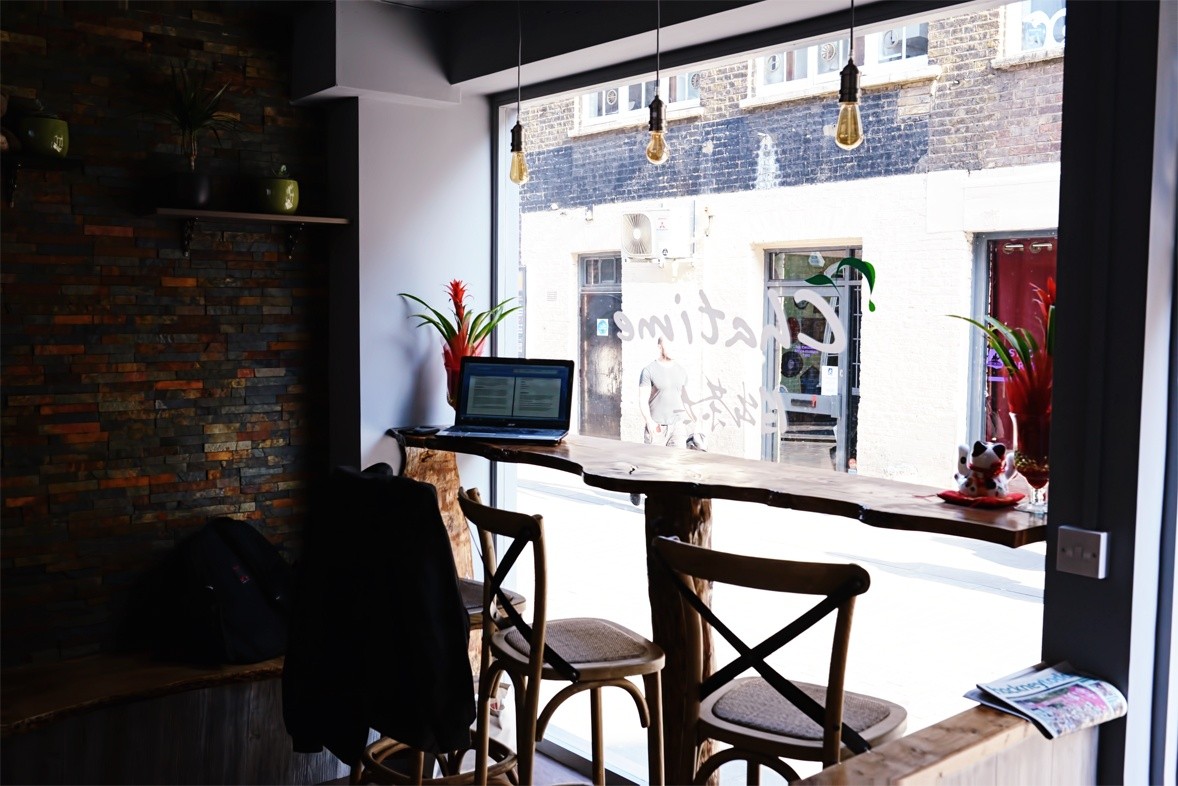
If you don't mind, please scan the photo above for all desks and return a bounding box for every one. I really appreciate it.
[389,423,1049,786]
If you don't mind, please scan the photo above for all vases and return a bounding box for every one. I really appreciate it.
[443,371,458,432]
[1008,412,1048,516]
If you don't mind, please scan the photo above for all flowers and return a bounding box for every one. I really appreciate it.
[393,279,525,368]
[939,277,1057,413]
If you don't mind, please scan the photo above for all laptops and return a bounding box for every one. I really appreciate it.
[434,356,574,445]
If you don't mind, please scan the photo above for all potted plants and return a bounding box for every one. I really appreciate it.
[271,163,301,211]
[20,97,69,158]
[157,53,239,206]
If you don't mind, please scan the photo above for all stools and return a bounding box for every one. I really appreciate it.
[659,534,909,786]
[456,486,667,786]
[284,460,517,786]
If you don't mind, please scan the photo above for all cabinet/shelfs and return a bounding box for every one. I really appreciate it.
[0,155,352,262]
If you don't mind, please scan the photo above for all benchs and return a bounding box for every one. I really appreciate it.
[0,647,336,786]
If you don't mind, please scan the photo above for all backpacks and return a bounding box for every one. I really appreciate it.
[172,518,290,670]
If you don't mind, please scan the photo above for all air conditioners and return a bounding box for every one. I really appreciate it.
[615,205,694,261]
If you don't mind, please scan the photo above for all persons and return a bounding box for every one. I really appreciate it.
[801,354,822,395]
[631,335,699,507]
